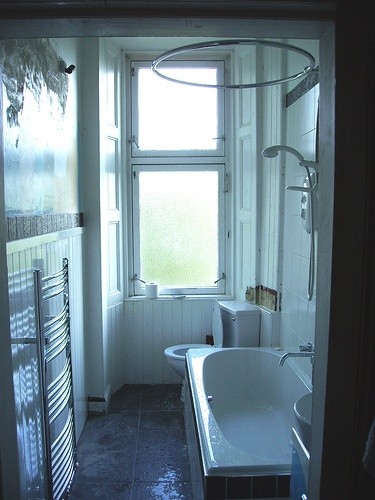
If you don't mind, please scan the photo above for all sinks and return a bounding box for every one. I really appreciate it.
[296,394,312,421]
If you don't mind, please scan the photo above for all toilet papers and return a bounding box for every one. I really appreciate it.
[146,283,158,299]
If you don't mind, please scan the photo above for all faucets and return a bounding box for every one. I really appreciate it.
[278,351,315,368]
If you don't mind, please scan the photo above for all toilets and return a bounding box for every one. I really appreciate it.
[163,300,262,404]
